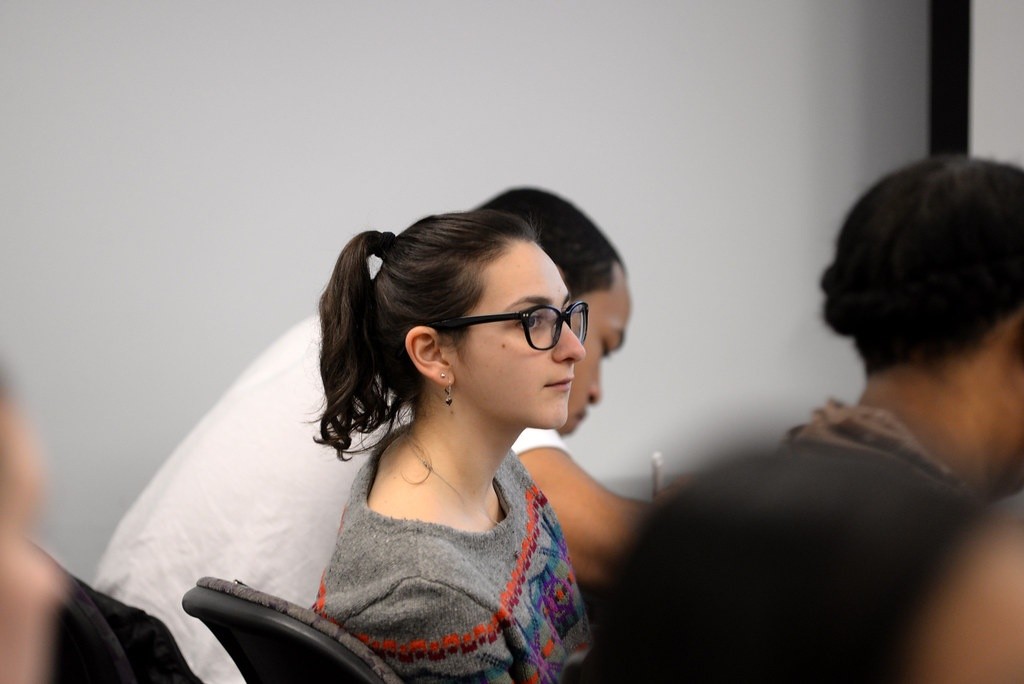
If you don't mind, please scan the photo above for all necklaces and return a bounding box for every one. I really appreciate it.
[404,426,523,561]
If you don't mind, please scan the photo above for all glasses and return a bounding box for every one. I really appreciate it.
[432,301,588,350]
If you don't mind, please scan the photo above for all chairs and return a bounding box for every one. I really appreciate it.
[182,576,405,684]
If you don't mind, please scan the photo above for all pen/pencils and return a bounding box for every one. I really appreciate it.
[649,451,664,498]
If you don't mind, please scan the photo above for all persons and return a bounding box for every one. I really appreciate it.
[0,372,205,684]
[573,436,1023,684]
[775,149,1022,500]
[92,185,663,684]
[309,208,599,684]
